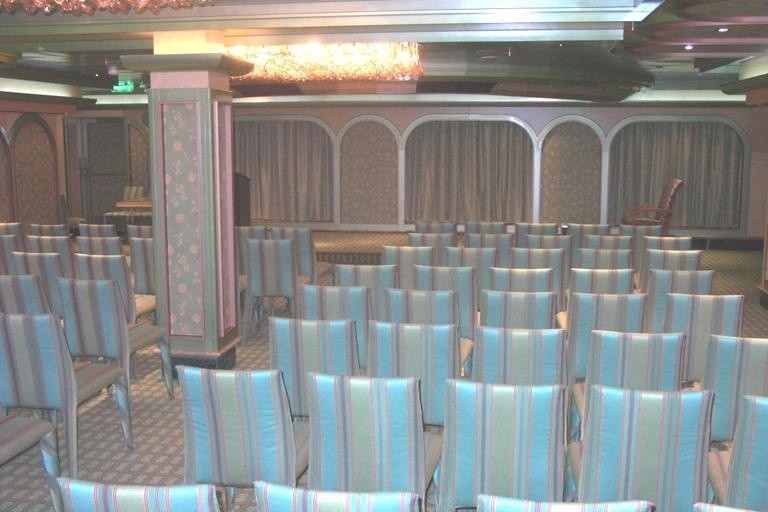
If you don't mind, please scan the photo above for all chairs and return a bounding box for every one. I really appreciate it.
[618,177,686,234]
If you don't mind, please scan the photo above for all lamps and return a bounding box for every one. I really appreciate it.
[227,36,423,81]
